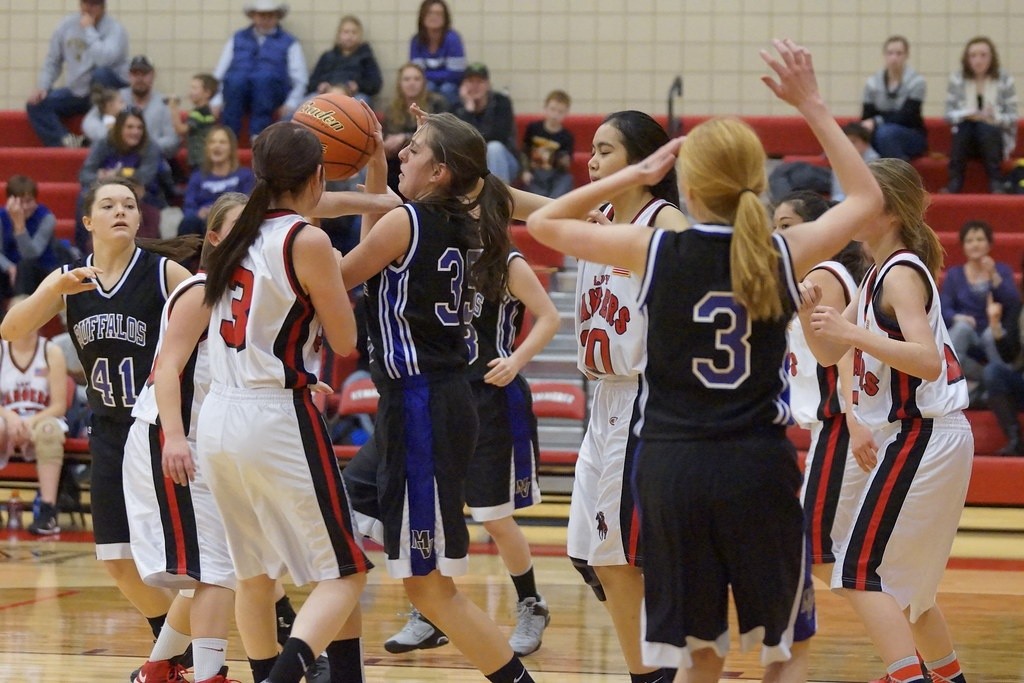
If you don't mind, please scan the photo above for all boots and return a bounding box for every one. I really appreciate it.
[990,394,1024,456]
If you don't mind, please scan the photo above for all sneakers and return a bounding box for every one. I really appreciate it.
[130,639,241,683]
[384,609,449,654]
[509,593,550,655]
[260,651,332,683]
[30,503,61,534]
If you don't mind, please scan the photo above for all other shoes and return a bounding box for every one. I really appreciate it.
[65,132,91,147]
[937,182,1009,192]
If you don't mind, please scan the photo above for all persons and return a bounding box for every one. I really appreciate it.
[0,0,1024,683]
[527,38,887,682]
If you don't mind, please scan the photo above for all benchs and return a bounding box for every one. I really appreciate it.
[0,106,1024,512]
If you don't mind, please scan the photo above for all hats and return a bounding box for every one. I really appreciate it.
[463,62,489,81]
[132,54,154,71]
[243,0,290,17]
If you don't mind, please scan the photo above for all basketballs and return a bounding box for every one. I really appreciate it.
[289,91,376,183]
